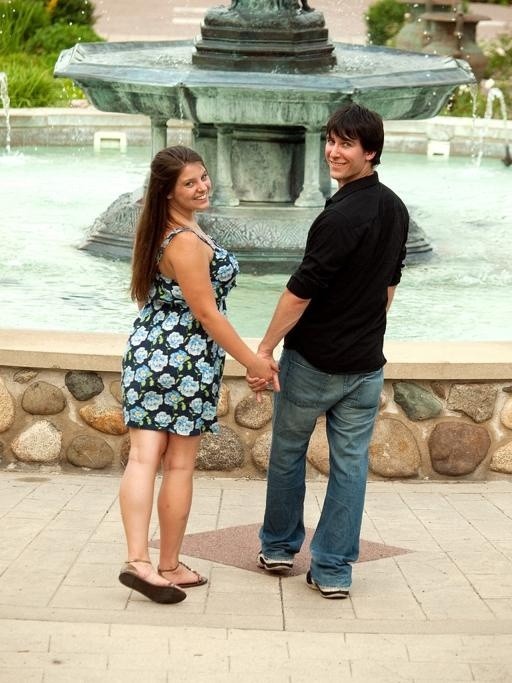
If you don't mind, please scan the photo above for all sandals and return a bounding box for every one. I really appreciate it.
[118,559,186,603]
[157,560,207,587]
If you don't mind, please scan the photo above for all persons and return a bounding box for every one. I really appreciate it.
[243,104,413,598]
[115,145,281,606]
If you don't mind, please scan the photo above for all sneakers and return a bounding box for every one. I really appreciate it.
[305,569,350,597]
[257,549,293,570]
